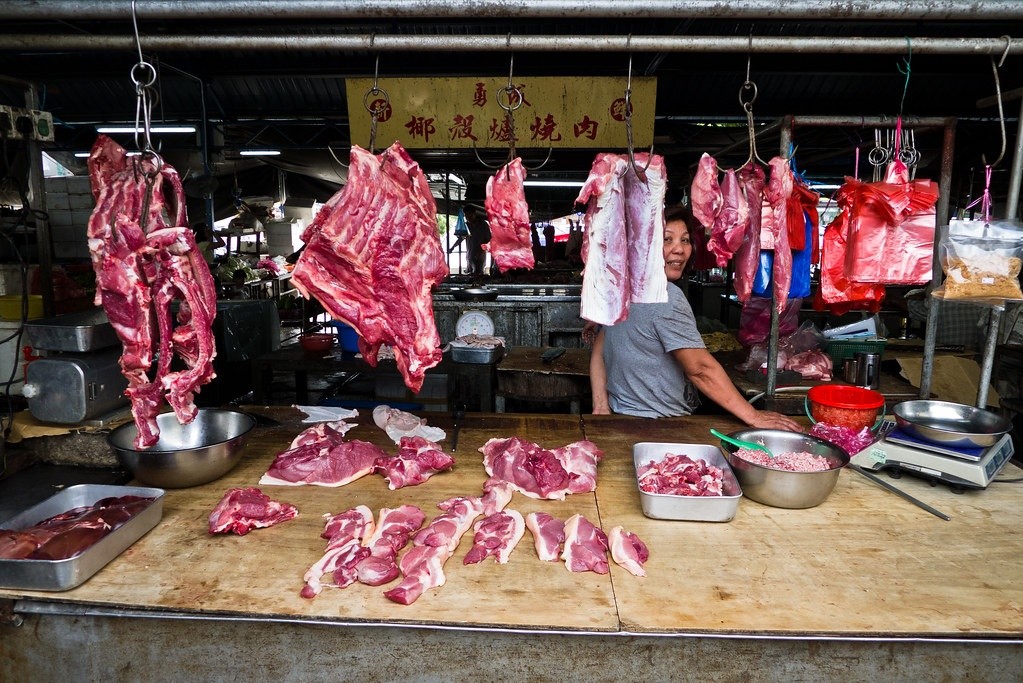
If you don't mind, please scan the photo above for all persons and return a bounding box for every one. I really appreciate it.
[449,205,491,275]
[193,222,225,264]
[582,321,598,344]
[228,269,248,299]
[589,205,806,433]
[886,292,981,346]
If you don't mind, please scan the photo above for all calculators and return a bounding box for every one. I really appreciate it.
[871,420,900,436]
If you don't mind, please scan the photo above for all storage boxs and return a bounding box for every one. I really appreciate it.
[825,336,889,368]
[375,372,449,411]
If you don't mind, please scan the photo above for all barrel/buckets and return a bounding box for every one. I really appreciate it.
[803,384,886,445]
[0,322,39,397]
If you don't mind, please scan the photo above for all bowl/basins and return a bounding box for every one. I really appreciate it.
[893,399,1013,448]
[105,406,256,490]
[721,429,851,509]
[331,320,360,353]
[0,294,43,320]
[298,334,334,350]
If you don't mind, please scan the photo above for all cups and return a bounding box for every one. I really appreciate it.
[841,359,857,383]
[855,352,881,389]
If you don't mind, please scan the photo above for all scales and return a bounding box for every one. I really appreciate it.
[850,423,1016,496]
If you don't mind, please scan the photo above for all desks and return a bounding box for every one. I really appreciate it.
[273,347,501,413]
